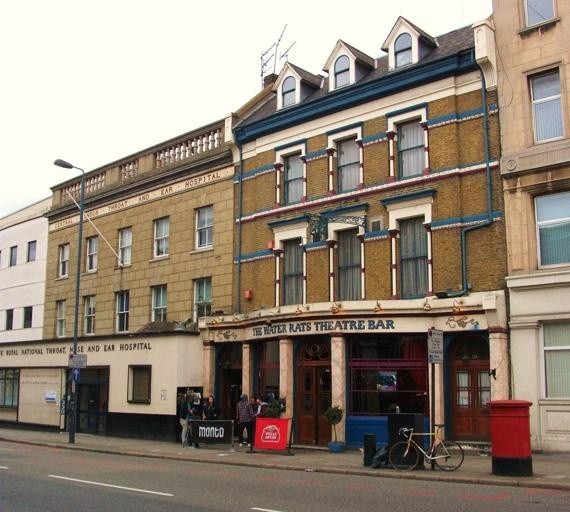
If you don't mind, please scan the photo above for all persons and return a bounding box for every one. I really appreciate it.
[203,395,218,420]
[179,395,194,447]
[256,396,269,417]
[249,396,258,414]
[236,394,252,447]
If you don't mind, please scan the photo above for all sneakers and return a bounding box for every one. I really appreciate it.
[239,443,243,448]
[247,443,251,447]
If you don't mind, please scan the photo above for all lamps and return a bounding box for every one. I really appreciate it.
[209,297,462,327]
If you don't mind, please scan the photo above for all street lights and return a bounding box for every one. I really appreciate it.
[52,156,87,448]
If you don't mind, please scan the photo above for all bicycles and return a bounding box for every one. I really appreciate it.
[389,423,464,471]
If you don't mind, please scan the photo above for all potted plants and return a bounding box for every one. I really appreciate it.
[324,403,346,453]
